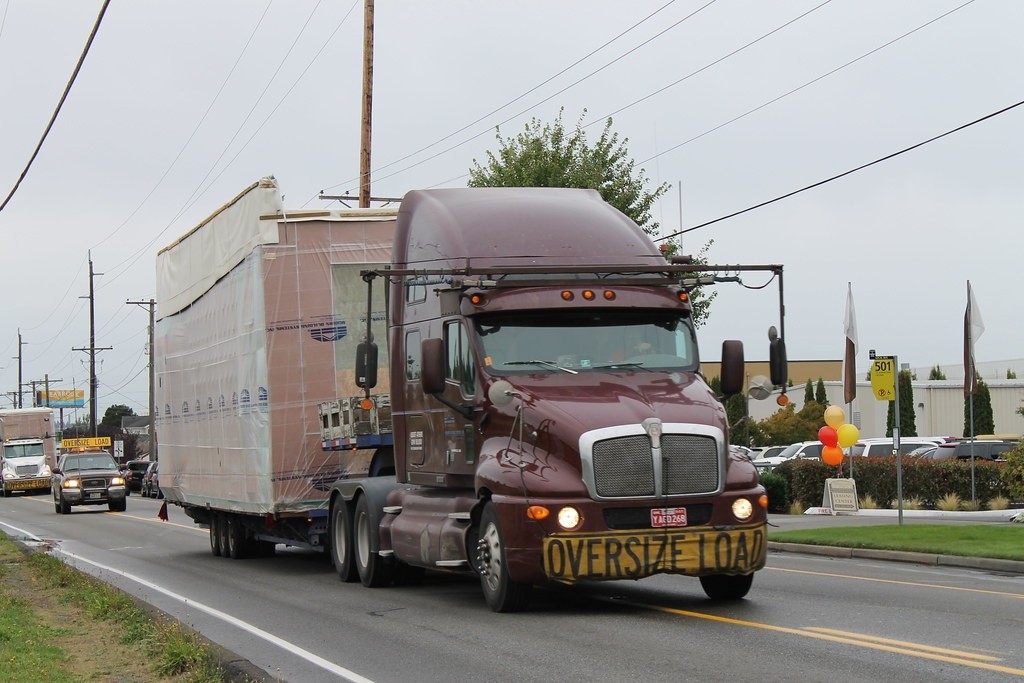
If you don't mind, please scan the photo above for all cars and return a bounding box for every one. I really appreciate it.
[730,433,1024,476]
[149,465,164,499]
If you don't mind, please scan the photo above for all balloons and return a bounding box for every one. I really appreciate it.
[824,406,844,430]
[837,424,858,449]
[818,426,837,449]
[821,444,844,466]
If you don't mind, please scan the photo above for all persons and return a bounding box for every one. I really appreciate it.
[612,326,652,363]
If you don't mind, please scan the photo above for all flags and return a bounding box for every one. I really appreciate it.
[963,284,984,398]
[844,285,859,405]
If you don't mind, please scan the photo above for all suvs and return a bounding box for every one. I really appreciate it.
[123,460,153,495]
[50,449,128,514]
[141,461,158,497]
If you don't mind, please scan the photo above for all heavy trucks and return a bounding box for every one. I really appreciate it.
[0,407,60,497]
[153,176,789,615]
